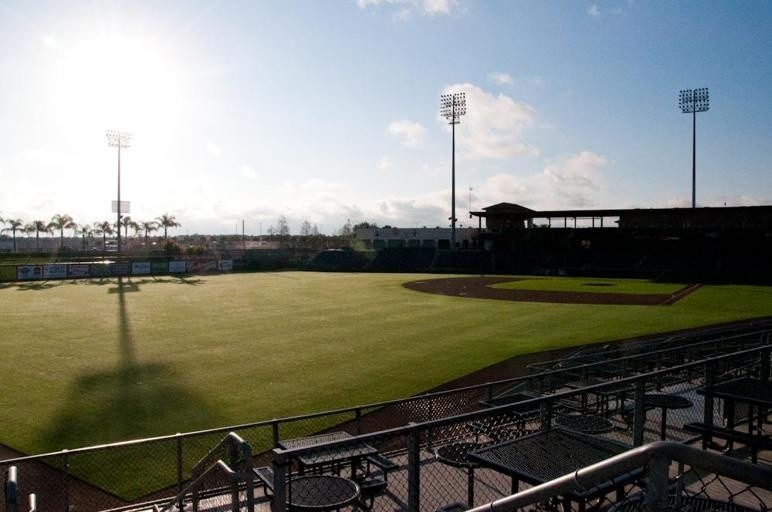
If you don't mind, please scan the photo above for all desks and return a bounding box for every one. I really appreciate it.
[697,377,772,463]
[480,391,561,436]
[277,430,378,483]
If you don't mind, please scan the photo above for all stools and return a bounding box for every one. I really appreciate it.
[641,392,693,441]
[436,444,493,509]
[559,411,615,436]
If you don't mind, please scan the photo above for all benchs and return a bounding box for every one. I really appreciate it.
[460,396,594,443]
[254,455,398,511]
[683,419,772,463]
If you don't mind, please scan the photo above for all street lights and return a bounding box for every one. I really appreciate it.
[677,88,709,207]
[104,126,134,286]
[441,93,467,249]
